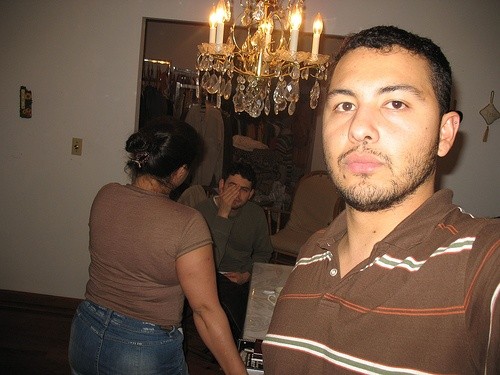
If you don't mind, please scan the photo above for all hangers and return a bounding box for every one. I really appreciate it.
[142,61,194,89]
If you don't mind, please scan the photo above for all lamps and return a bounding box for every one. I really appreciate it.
[194,0,330,118]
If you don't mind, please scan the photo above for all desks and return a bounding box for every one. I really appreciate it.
[238,262,295,355]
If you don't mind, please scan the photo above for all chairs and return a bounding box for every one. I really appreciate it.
[270,171,344,265]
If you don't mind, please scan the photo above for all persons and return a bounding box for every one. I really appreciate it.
[261,24,500,375]
[68,120,249,375]
[191,161,274,363]
